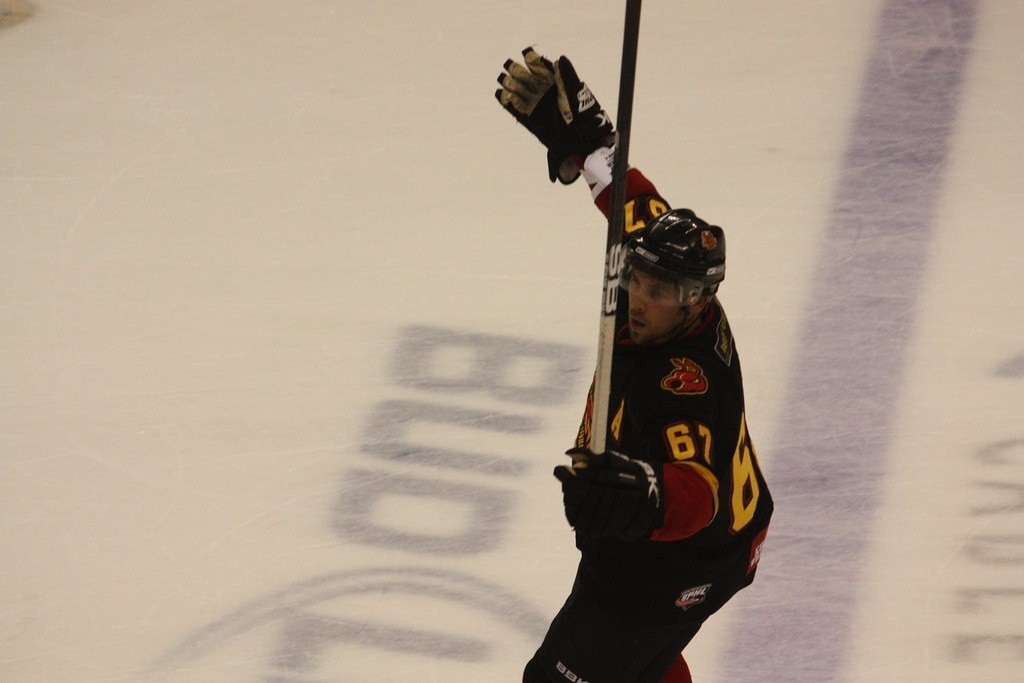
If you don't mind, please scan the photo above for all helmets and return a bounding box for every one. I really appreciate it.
[627,209,728,283]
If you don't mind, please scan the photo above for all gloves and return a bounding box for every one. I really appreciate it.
[554,445,668,543]
[495,46,617,186]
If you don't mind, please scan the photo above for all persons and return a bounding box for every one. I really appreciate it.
[494,45,776,683]
